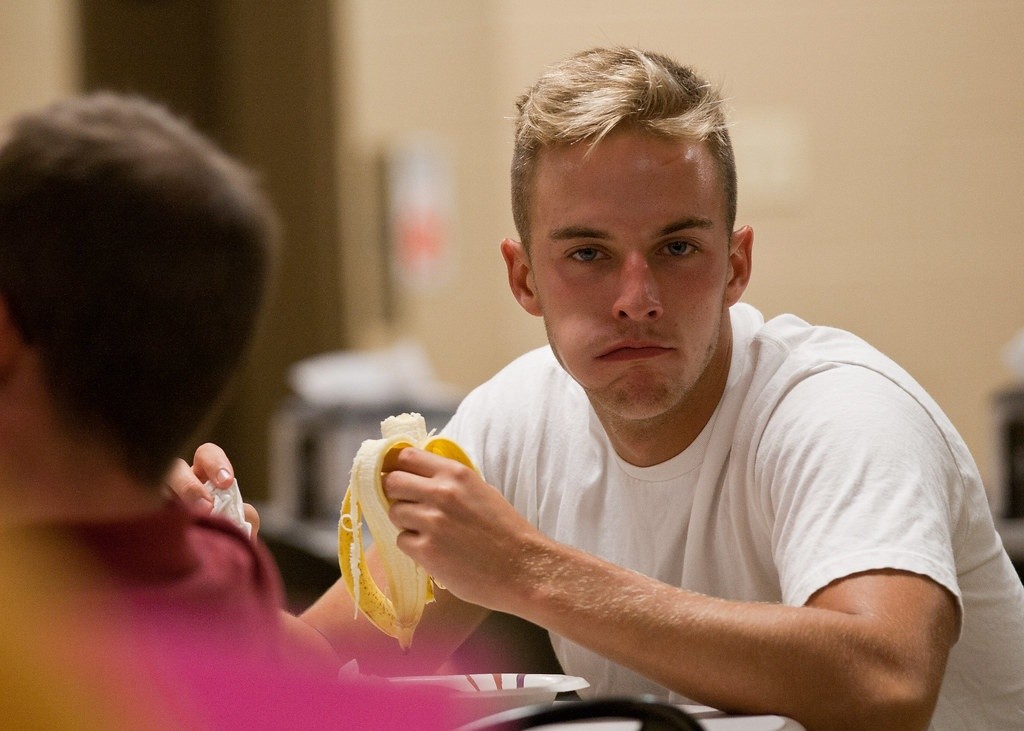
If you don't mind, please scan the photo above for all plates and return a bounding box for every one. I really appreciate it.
[376,673,591,713]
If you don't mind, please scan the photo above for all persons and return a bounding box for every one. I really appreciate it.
[0,90,278,731]
[156,50,1024,731]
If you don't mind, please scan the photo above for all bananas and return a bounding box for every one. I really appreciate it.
[337,411,486,653]
